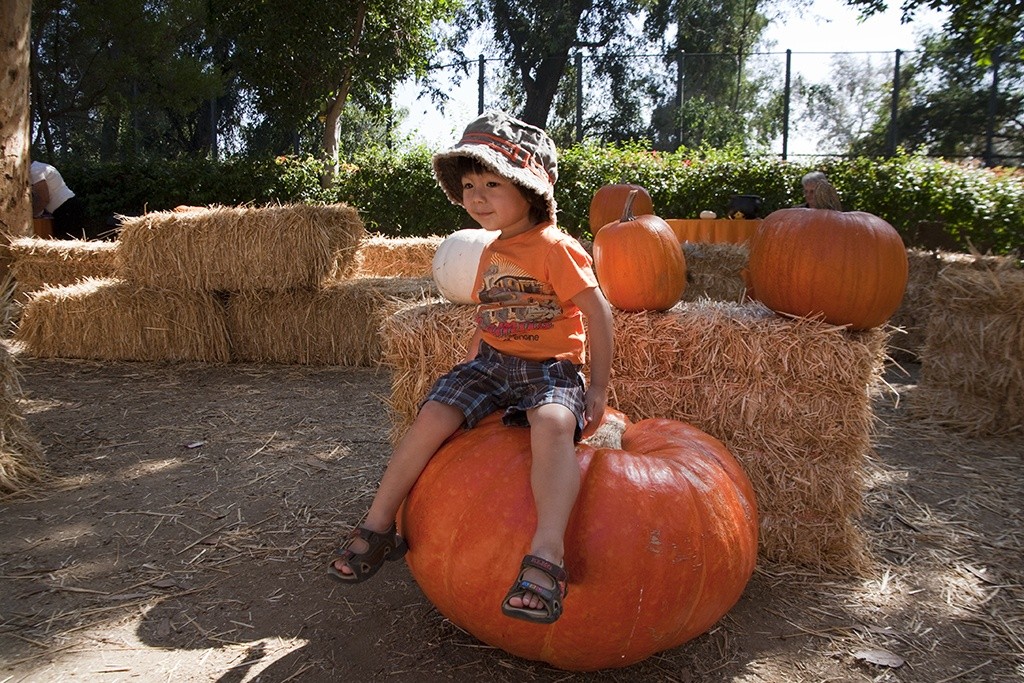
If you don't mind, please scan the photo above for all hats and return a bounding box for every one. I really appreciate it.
[432,110,559,225]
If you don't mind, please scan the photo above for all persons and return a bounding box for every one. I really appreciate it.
[790,170,828,208]
[28,158,85,241]
[323,109,614,624]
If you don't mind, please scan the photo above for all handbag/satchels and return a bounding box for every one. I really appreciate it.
[728,195,763,219]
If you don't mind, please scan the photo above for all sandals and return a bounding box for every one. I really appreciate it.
[327,521,408,584]
[501,555,569,623]
